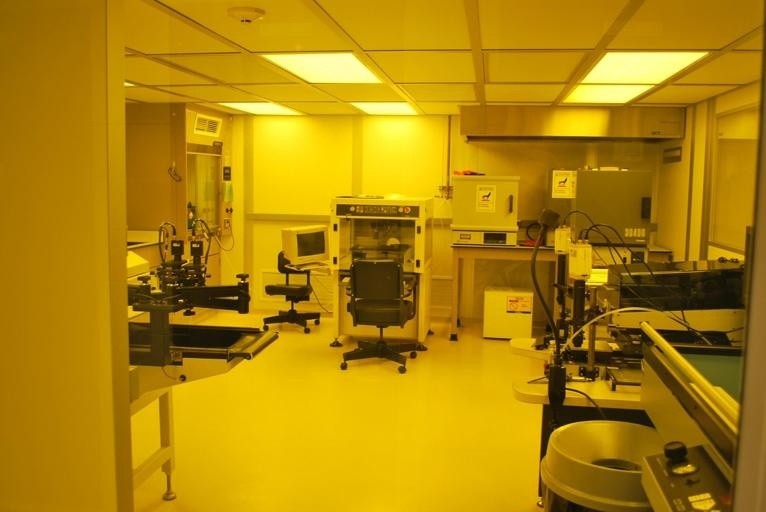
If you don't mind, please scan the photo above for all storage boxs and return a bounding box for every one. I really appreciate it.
[484,284,534,340]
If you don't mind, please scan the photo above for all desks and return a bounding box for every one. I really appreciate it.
[449,247,563,342]
[507,336,645,507]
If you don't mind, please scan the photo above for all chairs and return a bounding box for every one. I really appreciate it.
[263,251,321,334]
[340,261,418,374]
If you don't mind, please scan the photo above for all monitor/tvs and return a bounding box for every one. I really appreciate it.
[281,225,329,271]
